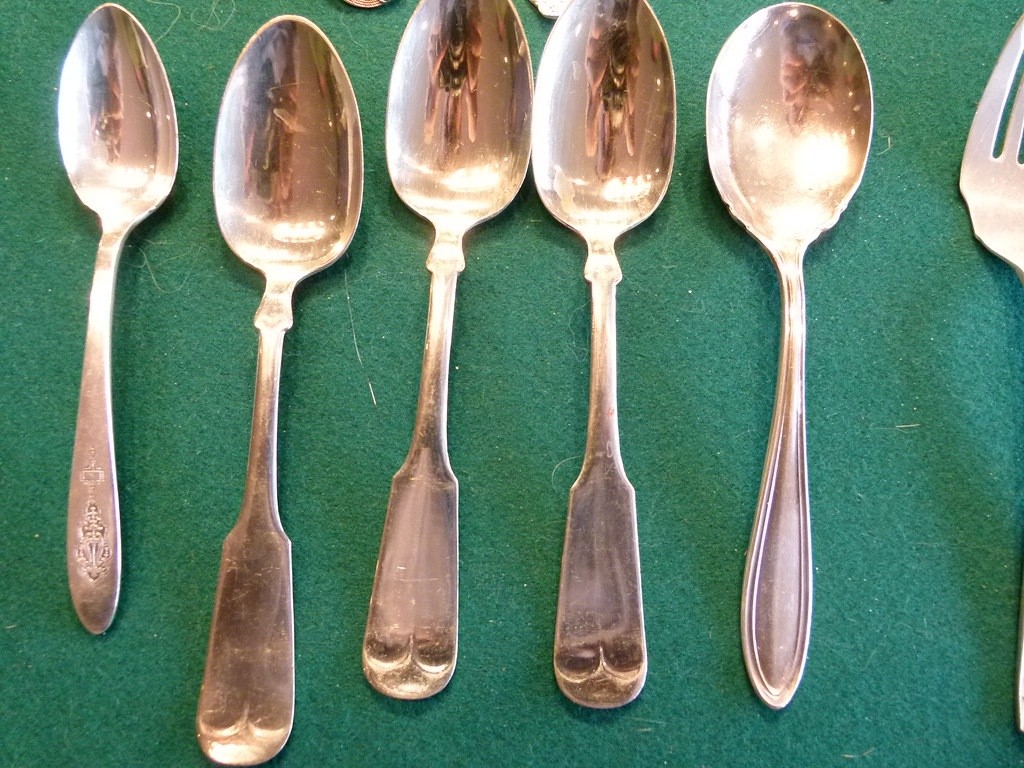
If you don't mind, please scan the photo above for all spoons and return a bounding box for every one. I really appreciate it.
[703,0,875,710]
[528,0,679,713]
[193,9,367,768]
[53,3,183,640]
[359,0,535,700]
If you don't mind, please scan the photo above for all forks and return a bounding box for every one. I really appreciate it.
[953,9,1024,277]
